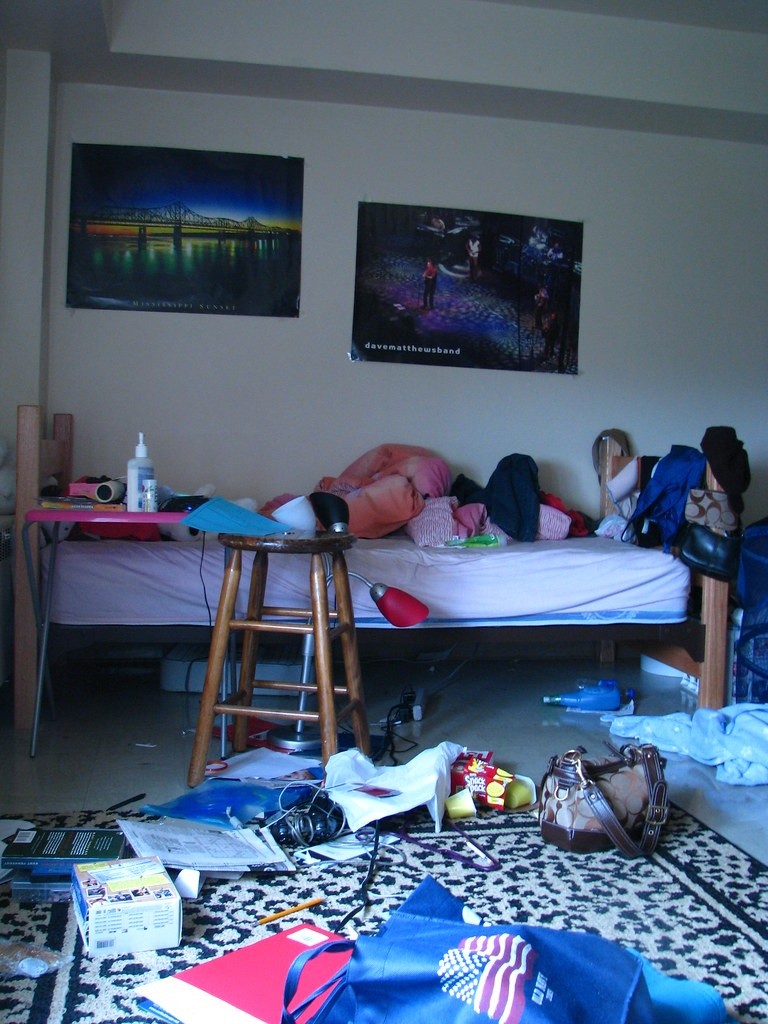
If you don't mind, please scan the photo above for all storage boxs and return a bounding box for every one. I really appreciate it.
[680,608,768,705]
[68,856,183,958]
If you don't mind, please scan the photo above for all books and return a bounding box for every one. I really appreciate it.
[10,868,73,904]
[0,828,125,871]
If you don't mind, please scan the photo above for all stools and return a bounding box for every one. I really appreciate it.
[187,528,370,787]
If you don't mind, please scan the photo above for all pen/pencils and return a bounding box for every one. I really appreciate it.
[464,839,495,866]
[105,791,148,812]
[251,896,327,928]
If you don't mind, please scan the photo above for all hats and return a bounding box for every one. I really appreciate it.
[700,427,751,513]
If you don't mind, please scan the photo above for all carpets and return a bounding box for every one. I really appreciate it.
[0,784,768,1024]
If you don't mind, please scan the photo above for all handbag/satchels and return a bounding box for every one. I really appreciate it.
[279,876,654,1024]
[679,521,744,582]
[539,740,668,858]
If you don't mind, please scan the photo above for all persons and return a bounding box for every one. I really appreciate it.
[421,216,565,355]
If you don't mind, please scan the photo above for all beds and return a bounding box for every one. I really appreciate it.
[15,404,740,762]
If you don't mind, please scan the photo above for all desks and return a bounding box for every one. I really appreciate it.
[21,506,240,759]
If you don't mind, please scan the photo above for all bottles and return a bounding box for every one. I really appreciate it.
[543,680,621,712]
[573,678,635,700]
[142,480,159,513]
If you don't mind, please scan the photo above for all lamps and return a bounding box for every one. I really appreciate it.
[269,488,434,751]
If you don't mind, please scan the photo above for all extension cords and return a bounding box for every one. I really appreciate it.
[413,687,428,720]
